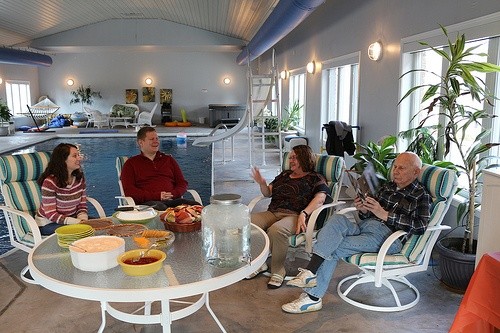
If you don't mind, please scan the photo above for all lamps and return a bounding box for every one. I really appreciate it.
[307,61,315,75]
[281,70,286,79]
[367,41,383,61]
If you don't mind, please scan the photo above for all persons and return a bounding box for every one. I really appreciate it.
[34,143,94,235]
[282,152,434,314]
[120,127,200,211]
[243,145,329,287]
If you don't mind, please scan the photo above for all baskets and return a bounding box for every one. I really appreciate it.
[160,212,201,233]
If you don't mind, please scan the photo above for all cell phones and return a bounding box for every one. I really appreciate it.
[115,207,134,211]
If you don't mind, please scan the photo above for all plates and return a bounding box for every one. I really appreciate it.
[55,224,95,248]
[135,229,176,248]
[79,219,113,230]
[106,223,147,236]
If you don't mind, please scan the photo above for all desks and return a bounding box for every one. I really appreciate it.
[275,130,299,148]
[209,103,246,127]
[474,169,500,273]
[28,211,269,333]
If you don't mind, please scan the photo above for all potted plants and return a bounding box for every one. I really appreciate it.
[396,21,500,294]
[0,103,13,136]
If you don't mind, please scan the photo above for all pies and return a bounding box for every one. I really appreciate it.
[79,218,114,230]
[106,223,147,238]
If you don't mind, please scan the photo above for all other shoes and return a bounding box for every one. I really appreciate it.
[267,268,286,289]
[245,262,268,280]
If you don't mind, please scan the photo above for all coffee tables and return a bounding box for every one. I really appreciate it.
[109,117,132,128]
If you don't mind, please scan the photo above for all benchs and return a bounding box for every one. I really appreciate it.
[111,104,140,127]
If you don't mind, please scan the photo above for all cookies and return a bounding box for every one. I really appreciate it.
[133,230,169,248]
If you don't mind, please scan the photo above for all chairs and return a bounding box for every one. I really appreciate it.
[84,107,110,128]
[114,156,203,211]
[138,104,158,126]
[336,163,459,313]
[0,151,105,284]
[248,152,346,282]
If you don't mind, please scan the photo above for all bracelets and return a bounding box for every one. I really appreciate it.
[303,211,309,218]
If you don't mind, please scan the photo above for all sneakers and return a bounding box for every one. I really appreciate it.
[281,293,323,314]
[287,267,318,288]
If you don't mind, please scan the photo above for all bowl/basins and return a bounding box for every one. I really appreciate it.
[118,249,166,276]
[69,236,125,272]
[160,213,201,233]
[113,209,158,225]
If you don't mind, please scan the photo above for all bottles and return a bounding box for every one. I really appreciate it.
[201,193,250,269]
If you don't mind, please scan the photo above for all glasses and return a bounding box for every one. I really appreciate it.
[289,156,298,160]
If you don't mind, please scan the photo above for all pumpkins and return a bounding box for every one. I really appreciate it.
[165,122,192,126]
